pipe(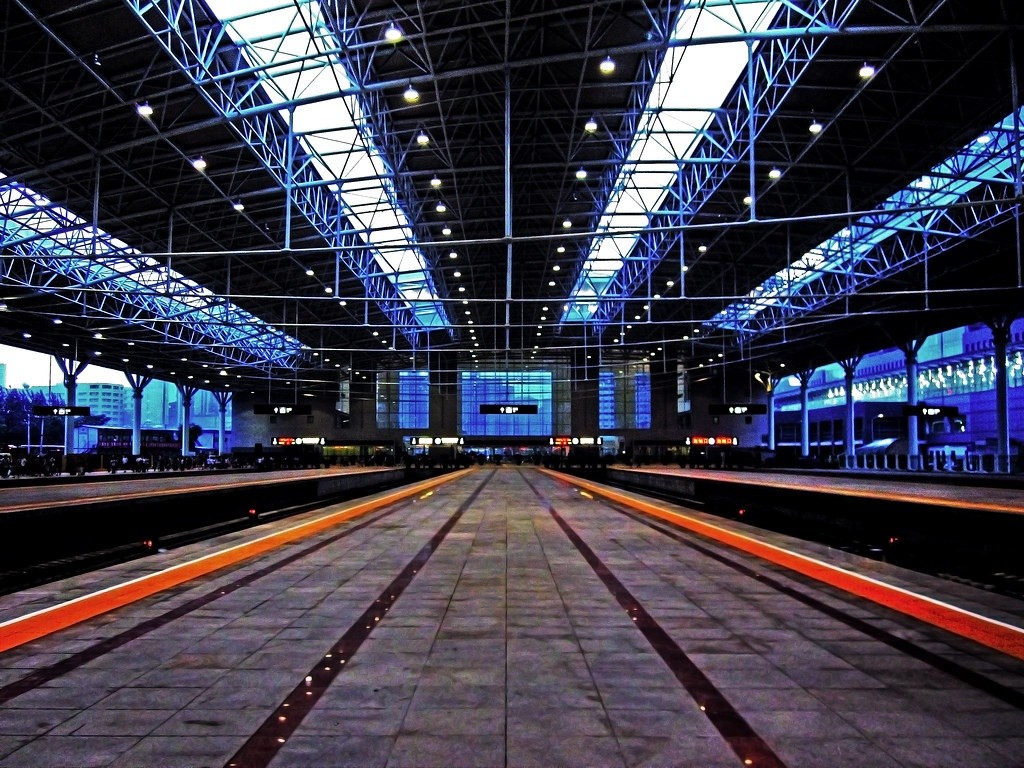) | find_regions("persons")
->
[0,442,740,476]
